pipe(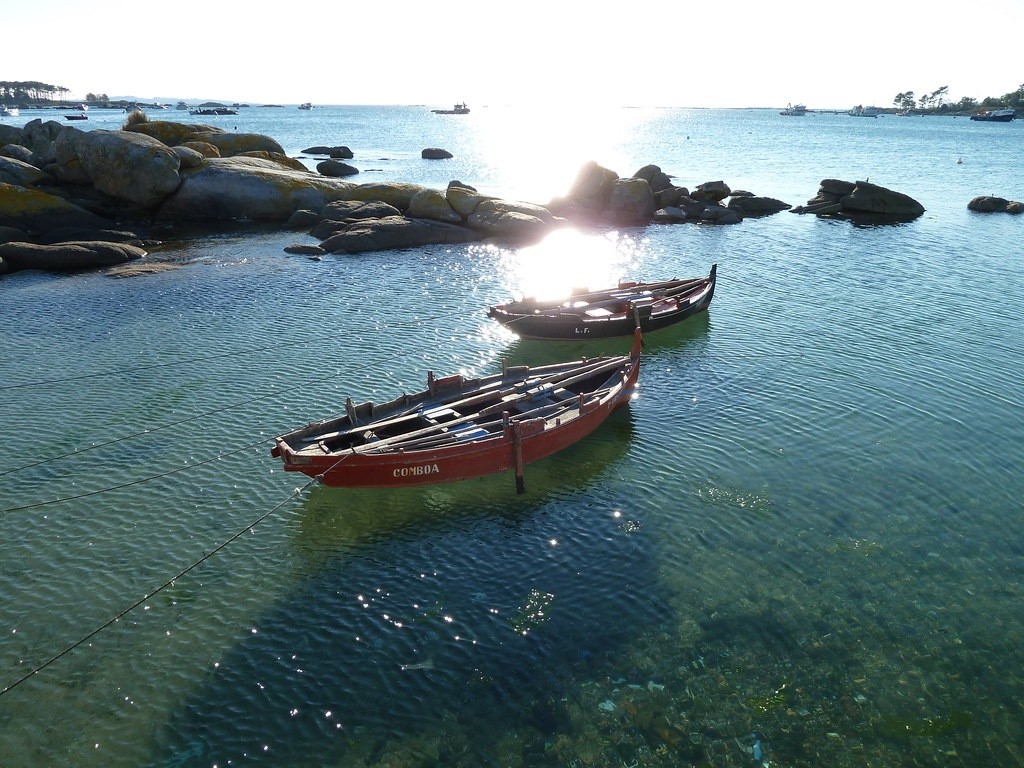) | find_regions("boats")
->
[272,326,643,489]
[0,104,19,116]
[487,264,720,339]
[188,105,239,115]
[430,102,470,114]
[970,109,1016,122]
[288,402,634,578]
[848,105,880,117]
[64,114,89,120]
[779,102,807,116]
